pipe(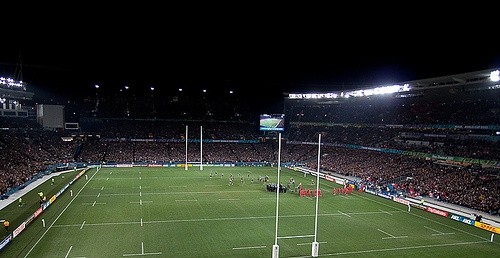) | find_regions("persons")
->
[4,219,10,233]
[0,97,500,217]
